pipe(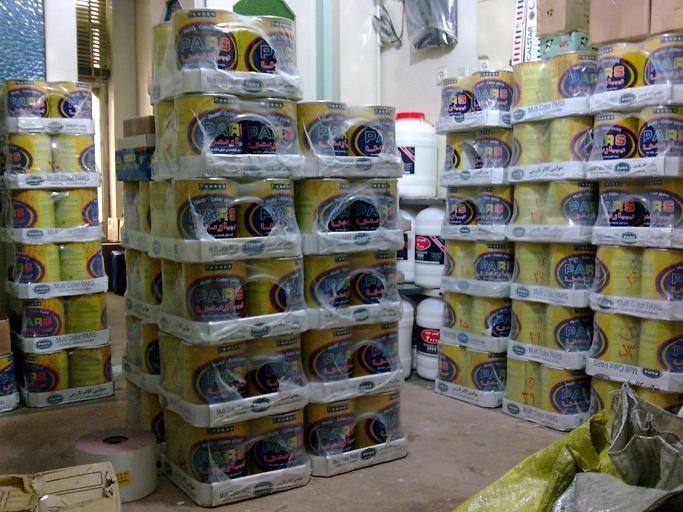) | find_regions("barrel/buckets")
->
[393,111,446,382]
